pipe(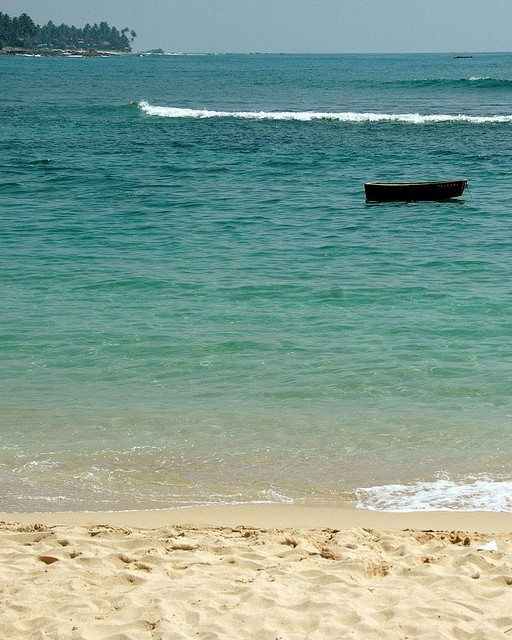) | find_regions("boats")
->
[365,180,465,202]
